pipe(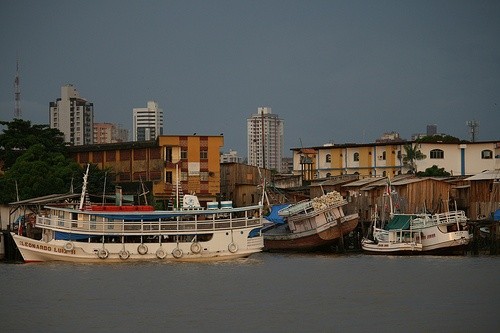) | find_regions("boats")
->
[360,197,474,254]
[260,191,358,250]
[9,161,270,261]
